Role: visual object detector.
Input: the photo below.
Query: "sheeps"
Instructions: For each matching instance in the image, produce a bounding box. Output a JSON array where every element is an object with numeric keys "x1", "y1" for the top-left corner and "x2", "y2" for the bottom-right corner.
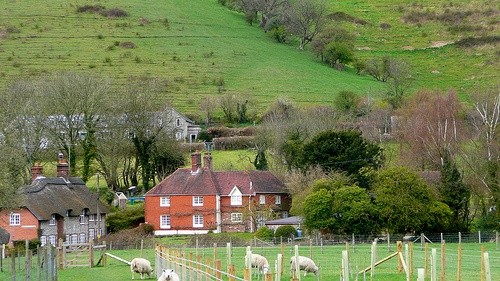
[
  {"x1": 290, "y1": 256, "x2": 319, "y2": 277},
  {"x1": 131, "y1": 257, "x2": 154, "y2": 279},
  {"x1": 245, "y1": 254, "x2": 269, "y2": 275},
  {"x1": 157, "y1": 269, "x2": 179, "y2": 281}
]
